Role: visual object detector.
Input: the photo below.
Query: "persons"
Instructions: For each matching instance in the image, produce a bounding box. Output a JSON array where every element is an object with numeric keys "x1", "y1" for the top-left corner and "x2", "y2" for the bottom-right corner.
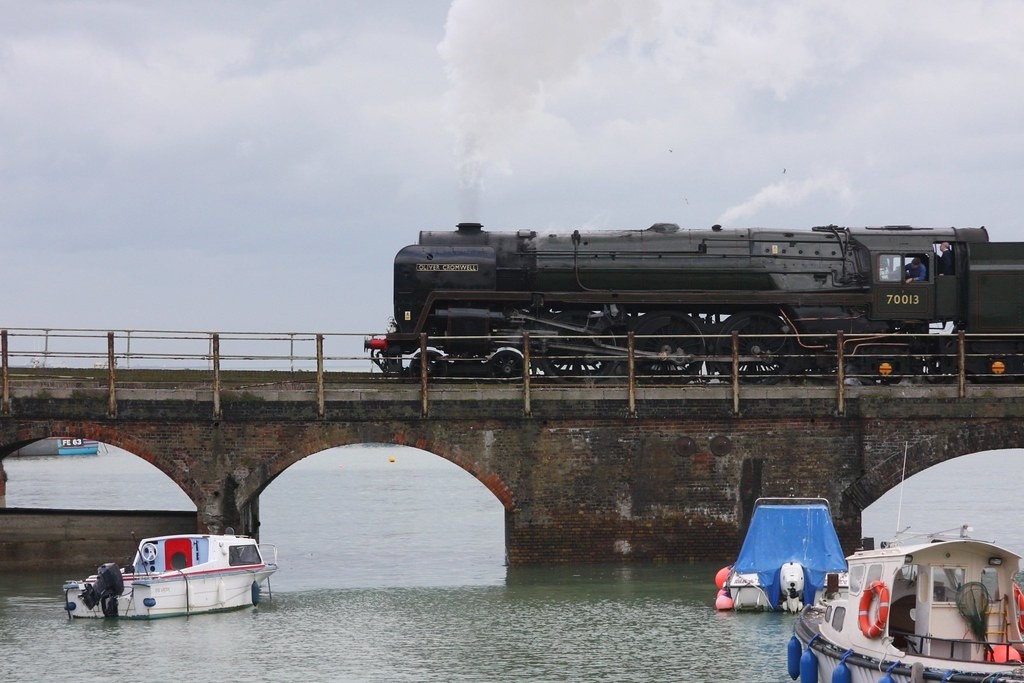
[
  {"x1": 904, "y1": 257, "x2": 926, "y2": 283},
  {"x1": 935, "y1": 242, "x2": 956, "y2": 276}
]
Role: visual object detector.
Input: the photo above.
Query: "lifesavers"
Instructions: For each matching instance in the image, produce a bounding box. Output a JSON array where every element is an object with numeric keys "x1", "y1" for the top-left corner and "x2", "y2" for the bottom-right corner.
[
  {"x1": 1014, "y1": 584, "x2": 1024, "y2": 635},
  {"x1": 859, "y1": 581, "x2": 889, "y2": 640}
]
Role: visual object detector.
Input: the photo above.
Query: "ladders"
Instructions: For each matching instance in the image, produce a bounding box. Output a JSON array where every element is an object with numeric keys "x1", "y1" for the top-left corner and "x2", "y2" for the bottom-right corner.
[{"x1": 981, "y1": 594, "x2": 1010, "y2": 651}]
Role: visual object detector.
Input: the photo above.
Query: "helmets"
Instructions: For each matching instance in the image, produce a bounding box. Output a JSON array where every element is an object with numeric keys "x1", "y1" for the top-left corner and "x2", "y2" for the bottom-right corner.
[
  {"x1": 715, "y1": 565, "x2": 733, "y2": 590},
  {"x1": 987, "y1": 644, "x2": 1022, "y2": 664}
]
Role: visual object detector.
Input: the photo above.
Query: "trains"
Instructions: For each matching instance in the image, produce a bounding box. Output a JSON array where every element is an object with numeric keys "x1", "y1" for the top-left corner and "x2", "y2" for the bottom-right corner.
[{"x1": 362, "y1": 222, "x2": 1024, "y2": 385}]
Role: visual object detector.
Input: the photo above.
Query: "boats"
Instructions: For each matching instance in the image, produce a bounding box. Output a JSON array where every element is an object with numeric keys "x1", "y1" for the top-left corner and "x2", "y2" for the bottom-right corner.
[
  {"x1": 61, "y1": 532, "x2": 277, "y2": 621},
  {"x1": 782, "y1": 441, "x2": 1024, "y2": 682},
  {"x1": 714, "y1": 497, "x2": 846, "y2": 611}
]
